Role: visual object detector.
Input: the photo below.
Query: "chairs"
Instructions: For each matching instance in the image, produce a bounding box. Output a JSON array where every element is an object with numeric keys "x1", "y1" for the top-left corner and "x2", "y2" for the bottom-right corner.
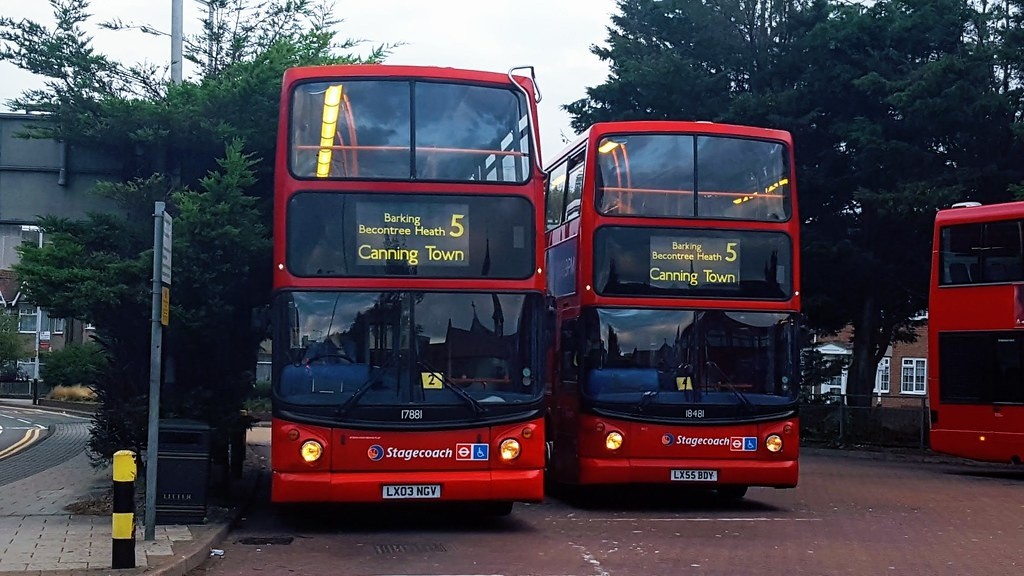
[
  {"x1": 949, "y1": 263, "x2": 971, "y2": 284},
  {"x1": 973, "y1": 265, "x2": 1003, "y2": 283}
]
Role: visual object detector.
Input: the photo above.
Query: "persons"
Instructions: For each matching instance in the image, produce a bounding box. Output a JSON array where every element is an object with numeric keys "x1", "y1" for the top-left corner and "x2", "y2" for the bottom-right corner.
[{"x1": 303, "y1": 316, "x2": 356, "y2": 364}]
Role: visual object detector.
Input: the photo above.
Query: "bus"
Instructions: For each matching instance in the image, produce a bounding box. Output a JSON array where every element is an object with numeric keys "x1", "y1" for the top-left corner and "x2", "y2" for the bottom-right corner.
[
  {"x1": 541, "y1": 118, "x2": 800, "y2": 498},
  {"x1": 271, "y1": 61, "x2": 547, "y2": 515},
  {"x1": 927, "y1": 199, "x2": 1024, "y2": 468}
]
